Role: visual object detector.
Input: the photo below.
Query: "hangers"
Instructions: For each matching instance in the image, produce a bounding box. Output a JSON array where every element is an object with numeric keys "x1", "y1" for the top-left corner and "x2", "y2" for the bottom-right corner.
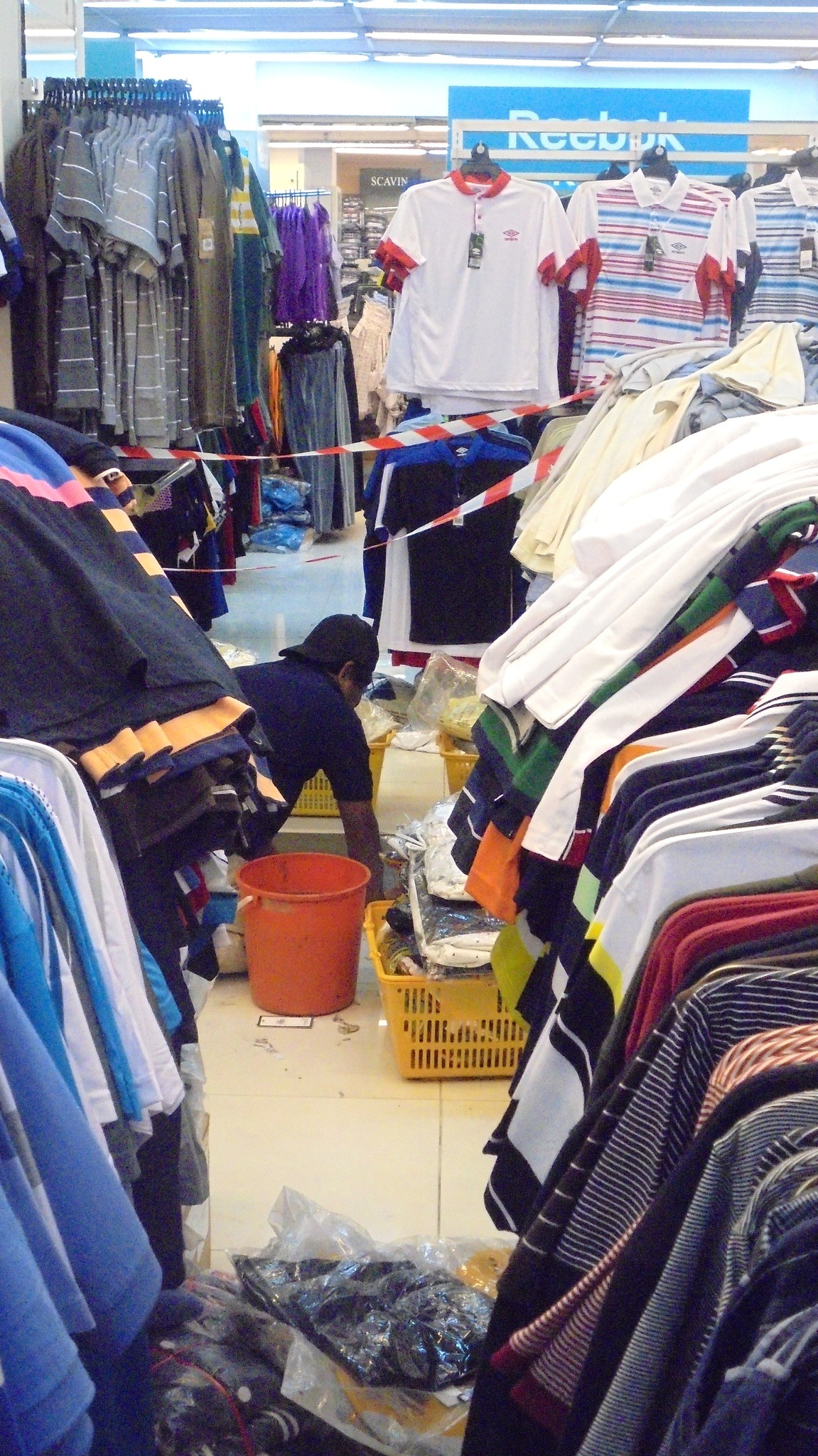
[
  {"x1": 0, "y1": 75, "x2": 818, "y2": 529},
  {"x1": 578, "y1": 581, "x2": 818, "y2": 1456}
]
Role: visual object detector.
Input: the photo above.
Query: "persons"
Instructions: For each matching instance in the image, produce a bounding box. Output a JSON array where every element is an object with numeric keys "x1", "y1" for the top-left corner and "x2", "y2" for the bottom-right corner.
[{"x1": 229, "y1": 614, "x2": 387, "y2": 908}]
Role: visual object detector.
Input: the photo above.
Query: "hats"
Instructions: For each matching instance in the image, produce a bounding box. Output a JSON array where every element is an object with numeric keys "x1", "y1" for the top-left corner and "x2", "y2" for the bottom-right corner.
[{"x1": 278, "y1": 613, "x2": 379, "y2": 668}]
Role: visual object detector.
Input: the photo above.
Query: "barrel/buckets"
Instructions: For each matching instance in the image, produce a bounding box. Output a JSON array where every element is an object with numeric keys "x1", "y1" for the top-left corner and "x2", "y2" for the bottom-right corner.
[{"x1": 235, "y1": 852, "x2": 372, "y2": 1017}]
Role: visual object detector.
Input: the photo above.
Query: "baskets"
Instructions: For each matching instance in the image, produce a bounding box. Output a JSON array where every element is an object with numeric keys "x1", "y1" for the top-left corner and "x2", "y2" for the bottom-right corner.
[
  {"x1": 364, "y1": 898, "x2": 532, "y2": 1080},
  {"x1": 441, "y1": 750, "x2": 484, "y2": 794},
  {"x1": 291, "y1": 729, "x2": 395, "y2": 819}
]
